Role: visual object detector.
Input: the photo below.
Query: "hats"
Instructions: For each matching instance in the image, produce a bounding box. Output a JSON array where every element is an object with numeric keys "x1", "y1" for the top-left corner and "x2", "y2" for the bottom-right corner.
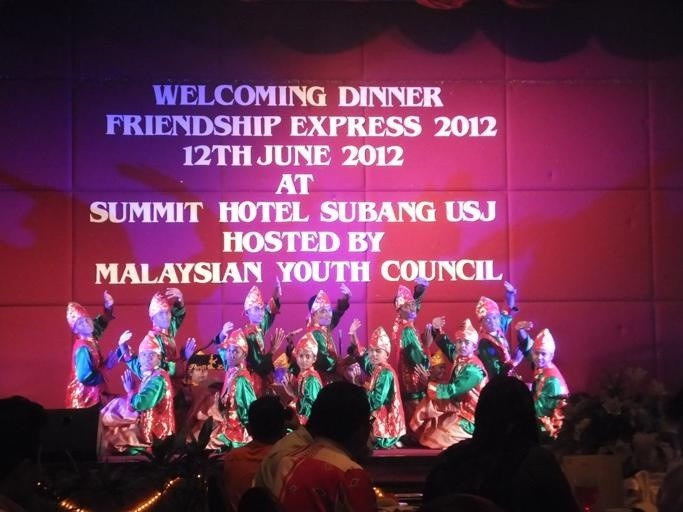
[
  {"x1": 395, "y1": 284, "x2": 415, "y2": 310},
  {"x1": 310, "y1": 289, "x2": 332, "y2": 314},
  {"x1": 295, "y1": 331, "x2": 320, "y2": 356},
  {"x1": 67, "y1": 302, "x2": 88, "y2": 329},
  {"x1": 455, "y1": 297, "x2": 499, "y2": 344},
  {"x1": 224, "y1": 329, "x2": 247, "y2": 354},
  {"x1": 243, "y1": 286, "x2": 265, "y2": 311},
  {"x1": 368, "y1": 328, "x2": 390, "y2": 355},
  {"x1": 531, "y1": 328, "x2": 555, "y2": 354},
  {"x1": 138, "y1": 292, "x2": 171, "y2": 355}
]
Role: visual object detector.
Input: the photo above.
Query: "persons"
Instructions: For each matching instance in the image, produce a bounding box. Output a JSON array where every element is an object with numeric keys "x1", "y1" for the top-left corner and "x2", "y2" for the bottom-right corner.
[
  {"x1": 655, "y1": 465, "x2": 683, "y2": 512},
  {"x1": 57, "y1": 274, "x2": 582, "y2": 512},
  {"x1": 0, "y1": 392, "x2": 50, "y2": 512}
]
